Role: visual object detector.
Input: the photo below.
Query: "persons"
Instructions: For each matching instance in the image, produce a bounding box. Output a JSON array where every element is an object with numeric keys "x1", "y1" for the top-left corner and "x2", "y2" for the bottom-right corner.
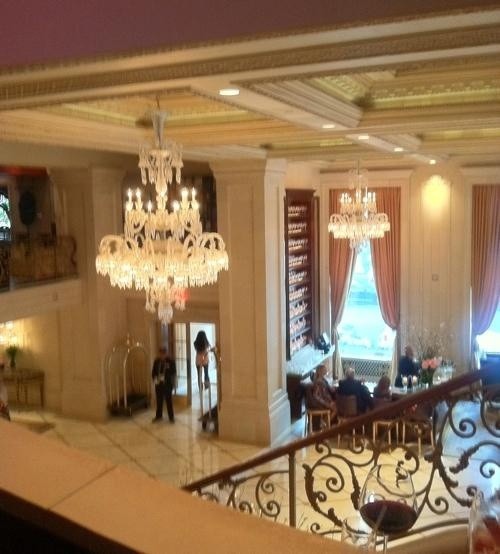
[
  {"x1": 307, "y1": 365, "x2": 338, "y2": 430},
  {"x1": 374, "y1": 375, "x2": 393, "y2": 398},
  {"x1": 334, "y1": 367, "x2": 371, "y2": 418},
  {"x1": 151, "y1": 345, "x2": 177, "y2": 424},
  {"x1": 395, "y1": 344, "x2": 431, "y2": 387},
  {"x1": 193, "y1": 330, "x2": 211, "y2": 392}
]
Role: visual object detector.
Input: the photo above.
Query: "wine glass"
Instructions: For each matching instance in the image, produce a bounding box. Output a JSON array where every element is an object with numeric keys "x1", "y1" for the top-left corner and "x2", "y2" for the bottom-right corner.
[{"x1": 358, "y1": 464, "x2": 419, "y2": 554}]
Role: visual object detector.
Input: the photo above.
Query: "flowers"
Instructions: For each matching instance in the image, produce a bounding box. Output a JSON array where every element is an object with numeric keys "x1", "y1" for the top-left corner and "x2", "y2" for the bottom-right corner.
[{"x1": 407, "y1": 320, "x2": 455, "y2": 382}]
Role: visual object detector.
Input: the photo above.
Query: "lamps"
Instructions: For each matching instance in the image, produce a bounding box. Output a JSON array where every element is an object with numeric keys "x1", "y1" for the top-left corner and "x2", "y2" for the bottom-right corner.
[
  {"x1": 326, "y1": 160, "x2": 391, "y2": 254},
  {"x1": 94, "y1": 93, "x2": 229, "y2": 326}
]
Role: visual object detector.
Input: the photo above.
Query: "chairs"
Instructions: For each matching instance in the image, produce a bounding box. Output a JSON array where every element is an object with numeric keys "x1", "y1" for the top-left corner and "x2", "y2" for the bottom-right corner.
[{"x1": 303, "y1": 384, "x2": 435, "y2": 460}]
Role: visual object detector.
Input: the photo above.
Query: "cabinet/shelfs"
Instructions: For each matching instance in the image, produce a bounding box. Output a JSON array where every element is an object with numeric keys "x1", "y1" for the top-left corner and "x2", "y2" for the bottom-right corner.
[{"x1": 282, "y1": 187, "x2": 317, "y2": 358}]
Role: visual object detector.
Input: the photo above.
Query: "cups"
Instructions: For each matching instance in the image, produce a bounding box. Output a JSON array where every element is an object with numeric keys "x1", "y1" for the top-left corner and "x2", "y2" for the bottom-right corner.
[
  {"x1": 341, "y1": 516, "x2": 377, "y2": 554},
  {"x1": 468, "y1": 487, "x2": 500, "y2": 554}
]
